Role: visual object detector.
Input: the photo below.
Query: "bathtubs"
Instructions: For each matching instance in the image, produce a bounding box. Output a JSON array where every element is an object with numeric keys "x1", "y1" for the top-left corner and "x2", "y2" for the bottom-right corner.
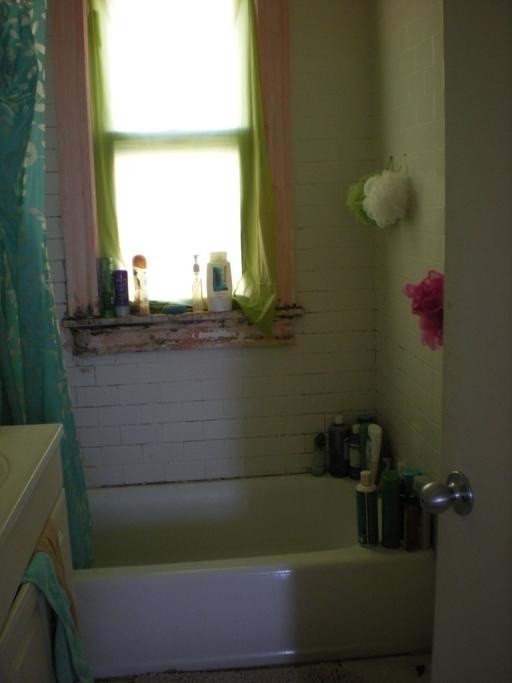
[{"x1": 72, "y1": 469, "x2": 436, "y2": 682}]
